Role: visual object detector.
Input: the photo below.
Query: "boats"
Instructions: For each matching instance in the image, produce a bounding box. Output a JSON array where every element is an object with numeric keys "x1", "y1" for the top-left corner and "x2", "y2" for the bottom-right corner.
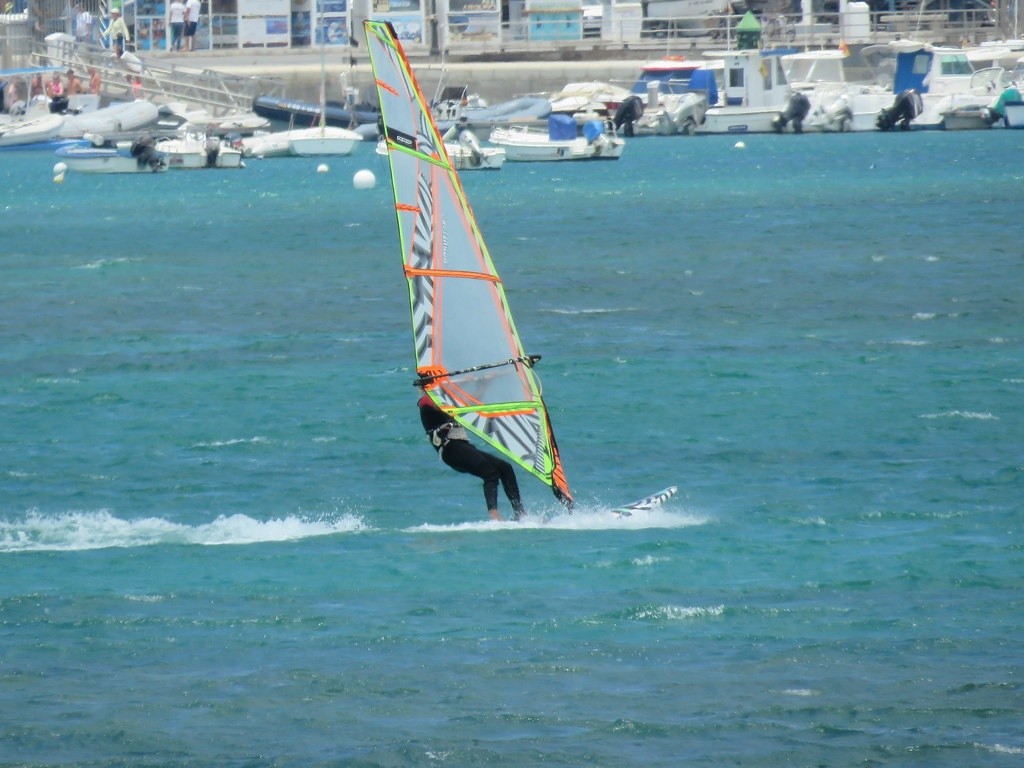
[
  {"x1": 54, "y1": 132, "x2": 172, "y2": 172},
  {"x1": 252, "y1": 84, "x2": 552, "y2": 133},
  {"x1": 154, "y1": 133, "x2": 243, "y2": 170},
  {"x1": 549, "y1": 33, "x2": 1024, "y2": 136},
  {"x1": 0, "y1": 95, "x2": 270, "y2": 147},
  {"x1": 489, "y1": 115, "x2": 624, "y2": 161},
  {"x1": 376, "y1": 137, "x2": 505, "y2": 170}
]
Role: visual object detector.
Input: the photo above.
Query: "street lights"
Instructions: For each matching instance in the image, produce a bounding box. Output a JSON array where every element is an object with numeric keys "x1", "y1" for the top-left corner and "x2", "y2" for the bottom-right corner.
[{"x1": 237, "y1": 1, "x2": 364, "y2": 157}]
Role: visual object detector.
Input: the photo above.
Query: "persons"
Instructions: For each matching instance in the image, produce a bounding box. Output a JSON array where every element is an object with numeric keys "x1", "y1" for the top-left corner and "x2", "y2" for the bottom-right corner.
[
  {"x1": 165, "y1": 0, "x2": 204, "y2": 52},
  {"x1": 0, "y1": 0, "x2": 141, "y2": 116},
  {"x1": 418, "y1": 379, "x2": 529, "y2": 525}
]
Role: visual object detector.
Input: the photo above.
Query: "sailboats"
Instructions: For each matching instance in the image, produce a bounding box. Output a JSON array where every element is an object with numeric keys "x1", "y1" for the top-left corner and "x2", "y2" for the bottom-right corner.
[{"x1": 363, "y1": 22, "x2": 679, "y2": 523}]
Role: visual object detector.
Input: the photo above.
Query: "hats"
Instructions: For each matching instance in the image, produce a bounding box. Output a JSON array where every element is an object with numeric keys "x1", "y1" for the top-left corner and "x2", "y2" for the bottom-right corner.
[{"x1": 110, "y1": 7, "x2": 119, "y2": 13}]
